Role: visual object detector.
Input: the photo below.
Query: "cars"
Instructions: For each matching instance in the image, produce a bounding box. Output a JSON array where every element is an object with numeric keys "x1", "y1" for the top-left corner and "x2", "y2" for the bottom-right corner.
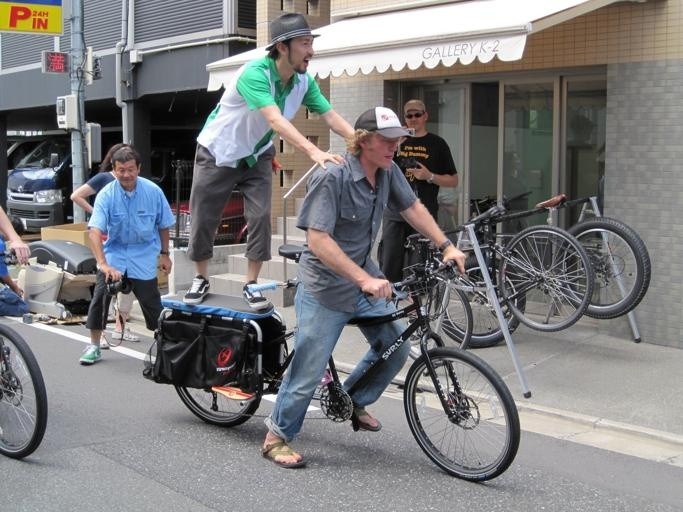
[{"x1": 167, "y1": 182, "x2": 249, "y2": 247}]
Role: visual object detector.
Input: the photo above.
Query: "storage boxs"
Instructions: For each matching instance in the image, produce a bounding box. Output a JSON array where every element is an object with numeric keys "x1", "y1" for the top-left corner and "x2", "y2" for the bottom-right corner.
[{"x1": 40, "y1": 222, "x2": 91, "y2": 248}]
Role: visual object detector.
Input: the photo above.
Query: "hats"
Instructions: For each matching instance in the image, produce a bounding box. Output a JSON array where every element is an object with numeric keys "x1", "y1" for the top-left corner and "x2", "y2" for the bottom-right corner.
[
  {"x1": 354, "y1": 106, "x2": 411, "y2": 139},
  {"x1": 403, "y1": 99, "x2": 425, "y2": 113},
  {"x1": 264, "y1": 12, "x2": 320, "y2": 50}
]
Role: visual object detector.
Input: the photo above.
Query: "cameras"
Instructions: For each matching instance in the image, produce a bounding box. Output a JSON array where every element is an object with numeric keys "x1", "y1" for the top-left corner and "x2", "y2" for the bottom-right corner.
[
  {"x1": 106, "y1": 275, "x2": 135, "y2": 294},
  {"x1": 405, "y1": 128, "x2": 415, "y2": 137}
]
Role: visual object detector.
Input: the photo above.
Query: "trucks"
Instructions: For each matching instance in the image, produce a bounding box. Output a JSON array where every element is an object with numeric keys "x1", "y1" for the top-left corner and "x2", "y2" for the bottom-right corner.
[
  {"x1": 5, "y1": 136, "x2": 59, "y2": 173},
  {"x1": 2, "y1": 136, "x2": 207, "y2": 233}
]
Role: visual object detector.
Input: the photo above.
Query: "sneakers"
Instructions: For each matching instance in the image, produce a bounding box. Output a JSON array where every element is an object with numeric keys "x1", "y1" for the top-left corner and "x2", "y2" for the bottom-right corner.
[
  {"x1": 182, "y1": 274, "x2": 210, "y2": 306},
  {"x1": 79, "y1": 345, "x2": 101, "y2": 364},
  {"x1": 99, "y1": 335, "x2": 109, "y2": 349},
  {"x1": 242, "y1": 280, "x2": 268, "y2": 311},
  {"x1": 111, "y1": 331, "x2": 140, "y2": 342},
  {"x1": 0, "y1": 360, "x2": 17, "y2": 387}
]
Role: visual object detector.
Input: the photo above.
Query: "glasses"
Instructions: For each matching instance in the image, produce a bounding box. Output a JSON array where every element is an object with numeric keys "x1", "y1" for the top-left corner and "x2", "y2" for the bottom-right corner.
[{"x1": 405, "y1": 111, "x2": 424, "y2": 118}]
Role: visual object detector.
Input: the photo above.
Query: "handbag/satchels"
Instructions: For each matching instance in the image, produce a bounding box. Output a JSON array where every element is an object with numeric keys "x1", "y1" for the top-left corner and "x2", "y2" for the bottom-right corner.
[{"x1": 143, "y1": 316, "x2": 249, "y2": 393}]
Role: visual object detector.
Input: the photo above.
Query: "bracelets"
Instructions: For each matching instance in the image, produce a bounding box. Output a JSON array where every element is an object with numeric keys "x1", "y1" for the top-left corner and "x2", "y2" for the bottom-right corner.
[
  {"x1": 439, "y1": 239, "x2": 452, "y2": 253},
  {"x1": 160, "y1": 250, "x2": 170, "y2": 256}
]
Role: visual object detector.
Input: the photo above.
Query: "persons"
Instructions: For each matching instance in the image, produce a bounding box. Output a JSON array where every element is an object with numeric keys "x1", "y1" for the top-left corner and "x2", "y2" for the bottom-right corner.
[
  {"x1": 79, "y1": 149, "x2": 175, "y2": 364},
  {"x1": 70, "y1": 144, "x2": 141, "y2": 349},
  {"x1": 378, "y1": 100, "x2": 459, "y2": 345},
  {"x1": 260, "y1": 107, "x2": 467, "y2": 469},
  {"x1": 1, "y1": 208, "x2": 30, "y2": 266},
  {"x1": 0, "y1": 215, "x2": 24, "y2": 297},
  {"x1": 182, "y1": 12, "x2": 358, "y2": 311}
]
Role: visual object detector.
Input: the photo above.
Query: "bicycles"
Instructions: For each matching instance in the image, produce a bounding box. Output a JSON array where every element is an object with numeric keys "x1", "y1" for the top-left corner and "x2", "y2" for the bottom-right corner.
[
  {"x1": 156, "y1": 244, "x2": 520, "y2": 483},
  {"x1": 393, "y1": 205, "x2": 594, "y2": 366},
  {"x1": 0, "y1": 248, "x2": 47, "y2": 459},
  {"x1": 433, "y1": 194, "x2": 652, "y2": 348}
]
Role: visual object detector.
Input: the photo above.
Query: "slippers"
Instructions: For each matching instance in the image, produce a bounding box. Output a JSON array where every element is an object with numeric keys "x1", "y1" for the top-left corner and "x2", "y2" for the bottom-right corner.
[
  {"x1": 260, "y1": 441, "x2": 307, "y2": 468},
  {"x1": 349, "y1": 407, "x2": 381, "y2": 431}
]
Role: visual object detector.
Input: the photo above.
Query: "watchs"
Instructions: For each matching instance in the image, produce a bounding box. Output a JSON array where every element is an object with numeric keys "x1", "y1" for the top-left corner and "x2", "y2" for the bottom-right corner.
[{"x1": 427, "y1": 173, "x2": 434, "y2": 184}]
